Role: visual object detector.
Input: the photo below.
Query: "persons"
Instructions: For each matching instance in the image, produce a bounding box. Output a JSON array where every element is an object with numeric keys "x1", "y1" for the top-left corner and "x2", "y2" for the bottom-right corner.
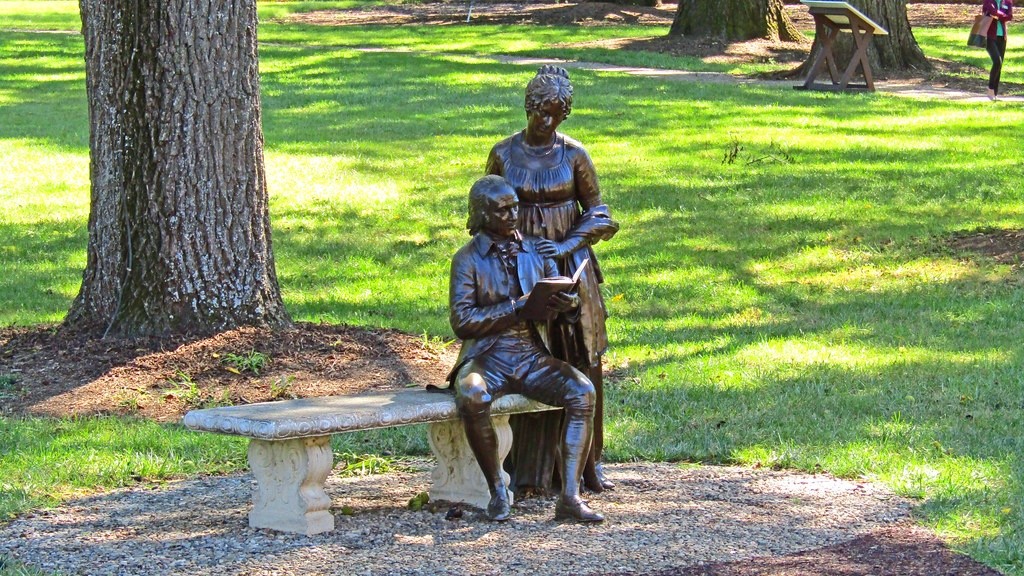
[
  {"x1": 449, "y1": 174, "x2": 610, "y2": 523},
  {"x1": 478, "y1": 65, "x2": 627, "y2": 491},
  {"x1": 981, "y1": 0, "x2": 1014, "y2": 101}
]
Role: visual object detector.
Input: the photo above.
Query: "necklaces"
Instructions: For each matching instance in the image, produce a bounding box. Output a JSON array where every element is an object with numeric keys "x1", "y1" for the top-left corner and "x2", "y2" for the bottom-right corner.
[{"x1": 518, "y1": 127, "x2": 559, "y2": 157}]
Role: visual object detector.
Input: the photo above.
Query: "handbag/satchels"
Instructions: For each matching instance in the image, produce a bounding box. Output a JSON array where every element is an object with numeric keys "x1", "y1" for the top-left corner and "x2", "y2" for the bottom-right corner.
[{"x1": 969, "y1": 8, "x2": 993, "y2": 49}]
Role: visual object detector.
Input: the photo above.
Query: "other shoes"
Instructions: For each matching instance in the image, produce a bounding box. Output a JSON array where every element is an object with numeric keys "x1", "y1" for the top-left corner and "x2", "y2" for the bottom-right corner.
[{"x1": 987, "y1": 87, "x2": 1002, "y2": 102}]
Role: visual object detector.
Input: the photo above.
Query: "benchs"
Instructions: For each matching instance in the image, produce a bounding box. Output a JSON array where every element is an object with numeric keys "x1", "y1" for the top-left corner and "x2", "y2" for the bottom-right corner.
[{"x1": 182, "y1": 383, "x2": 568, "y2": 538}]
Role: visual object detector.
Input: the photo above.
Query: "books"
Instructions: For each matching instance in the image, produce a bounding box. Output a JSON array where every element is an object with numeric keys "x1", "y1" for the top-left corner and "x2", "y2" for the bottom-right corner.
[{"x1": 516, "y1": 257, "x2": 592, "y2": 321}]
